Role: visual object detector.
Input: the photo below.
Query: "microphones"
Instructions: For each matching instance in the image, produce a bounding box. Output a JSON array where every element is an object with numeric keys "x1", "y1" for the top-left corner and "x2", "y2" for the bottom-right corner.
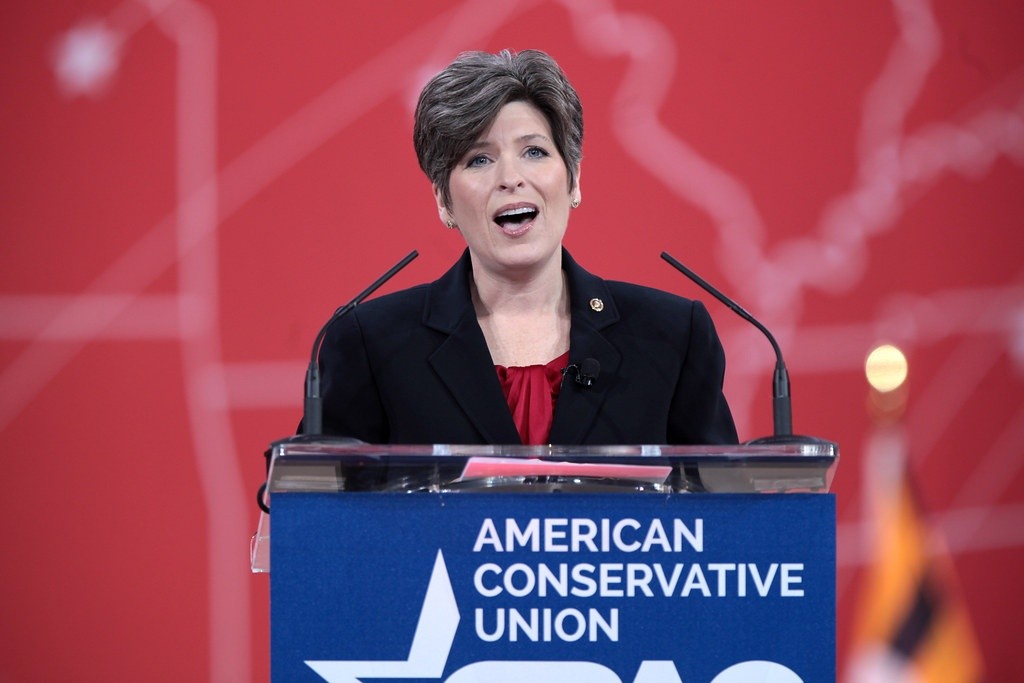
[
  {"x1": 581, "y1": 358, "x2": 601, "y2": 387},
  {"x1": 661, "y1": 251, "x2": 824, "y2": 448},
  {"x1": 269, "y1": 249, "x2": 419, "y2": 450}
]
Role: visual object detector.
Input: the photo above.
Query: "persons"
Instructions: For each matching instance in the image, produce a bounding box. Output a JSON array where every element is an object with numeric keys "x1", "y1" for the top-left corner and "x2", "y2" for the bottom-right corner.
[{"x1": 296, "y1": 47, "x2": 740, "y2": 493}]
